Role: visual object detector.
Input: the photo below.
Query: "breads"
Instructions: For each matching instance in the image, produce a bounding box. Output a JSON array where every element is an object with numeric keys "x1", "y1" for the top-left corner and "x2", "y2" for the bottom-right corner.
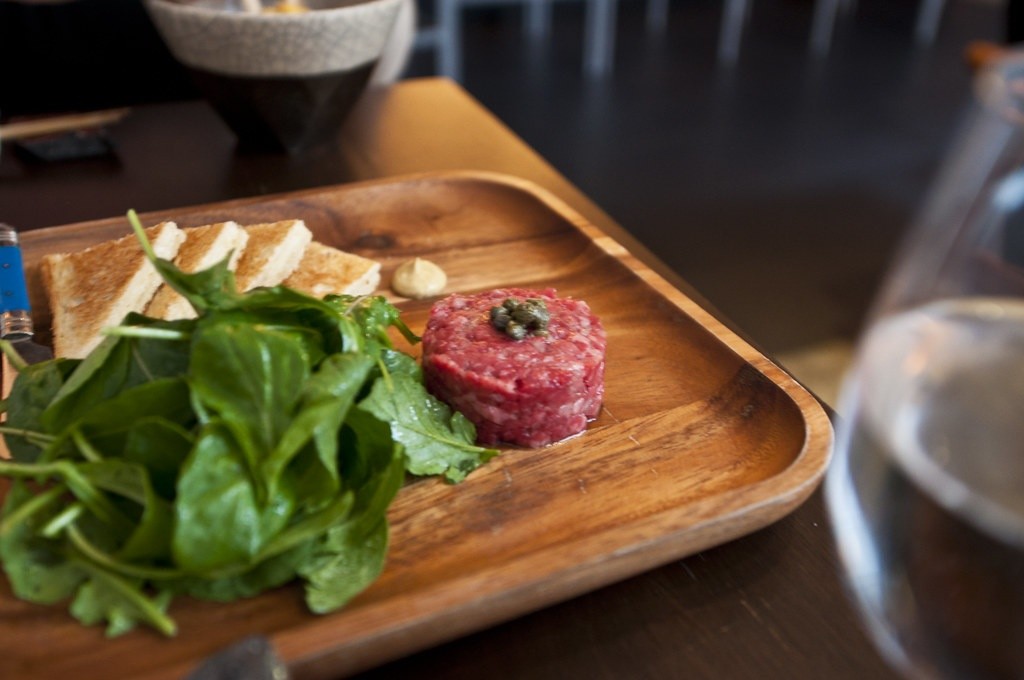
[{"x1": 39, "y1": 217, "x2": 382, "y2": 360}]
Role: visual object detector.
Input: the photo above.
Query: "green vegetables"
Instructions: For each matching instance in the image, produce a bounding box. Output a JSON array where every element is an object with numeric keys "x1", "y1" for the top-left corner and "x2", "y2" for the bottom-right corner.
[{"x1": 0, "y1": 208, "x2": 503, "y2": 638}]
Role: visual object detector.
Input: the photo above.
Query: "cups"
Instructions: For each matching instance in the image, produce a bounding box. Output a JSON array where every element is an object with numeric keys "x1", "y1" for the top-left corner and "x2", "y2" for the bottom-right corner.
[
  {"x1": 823, "y1": 58, "x2": 1024, "y2": 679},
  {"x1": 146, "y1": 0, "x2": 402, "y2": 157}
]
